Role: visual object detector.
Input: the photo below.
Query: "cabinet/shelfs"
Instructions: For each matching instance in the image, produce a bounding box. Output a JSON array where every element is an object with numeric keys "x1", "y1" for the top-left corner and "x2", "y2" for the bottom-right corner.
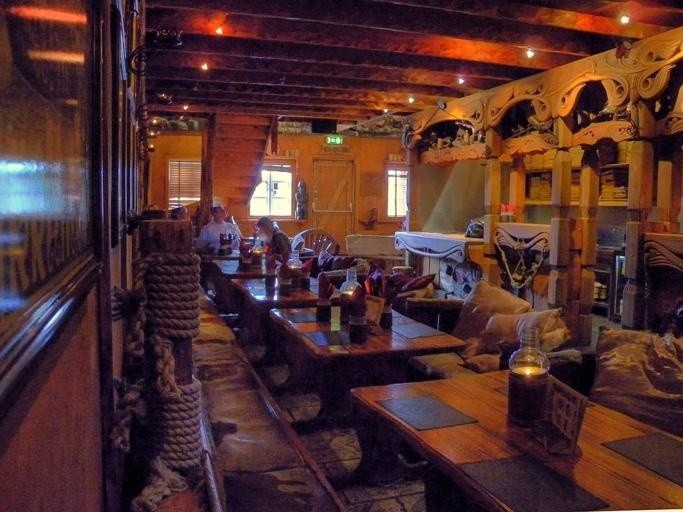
[{"x1": 508, "y1": 139, "x2": 653, "y2": 224}]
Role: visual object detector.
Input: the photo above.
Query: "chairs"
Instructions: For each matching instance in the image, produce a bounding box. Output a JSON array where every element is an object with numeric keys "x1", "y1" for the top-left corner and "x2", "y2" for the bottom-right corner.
[
  {"x1": 547, "y1": 345, "x2": 596, "y2": 398},
  {"x1": 299, "y1": 228, "x2": 339, "y2": 255},
  {"x1": 288, "y1": 235, "x2": 314, "y2": 256},
  {"x1": 299, "y1": 256, "x2": 385, "y2": 288},
  {"x1": 403, "y1": 298, "x2": 595, "y2": 397}
]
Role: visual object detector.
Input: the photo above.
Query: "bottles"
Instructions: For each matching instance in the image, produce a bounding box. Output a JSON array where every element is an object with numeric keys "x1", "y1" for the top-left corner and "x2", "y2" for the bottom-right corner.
[
  {"x1": 265, "y1": 250, "x2": 310, "y2": 295},
  {"x1": 219, "y1": 232, "x2": 263, "y2": 269},
  {"x1": 507, "y1": 326, "x2": 551, "y2": 427},
  {"x1": 463, "y1": 130, "x2": 469, "y2": 146},
  {"x1": 316, "y1": 267, "x2": 393, "y2": 344}
]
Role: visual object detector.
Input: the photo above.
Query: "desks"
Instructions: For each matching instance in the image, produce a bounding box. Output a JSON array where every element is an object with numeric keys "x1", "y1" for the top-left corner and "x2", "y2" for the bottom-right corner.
[
  {"x1": 268, "y1": 304, "x2": 466, "y2": 430},
  {"x1": 347, "y1": 368, "x2": 682, "y2": 512},
  {"x1": 203, "y1": 244, "x2": 242, "y2": 288},
  {"x1": 229, "y1": 275, "x2": 318, "y2": 344},
  {"x1": 210, "y1": 259, "x2": 270, "y2": 311},
  {"x1": 328, "y1": 271, "x2": 464, "y2": 334}
]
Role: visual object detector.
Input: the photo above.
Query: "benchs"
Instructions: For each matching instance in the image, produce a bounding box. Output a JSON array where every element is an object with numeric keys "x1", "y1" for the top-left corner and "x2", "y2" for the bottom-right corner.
[{"x1": 345, "y1": 233, "x2": 405, "y2": 273}]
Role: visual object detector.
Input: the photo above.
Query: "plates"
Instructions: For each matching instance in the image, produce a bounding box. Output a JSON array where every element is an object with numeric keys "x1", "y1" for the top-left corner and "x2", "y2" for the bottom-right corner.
[{"x1": 593, "y1": 281, "x2": 608, "y2": 300}]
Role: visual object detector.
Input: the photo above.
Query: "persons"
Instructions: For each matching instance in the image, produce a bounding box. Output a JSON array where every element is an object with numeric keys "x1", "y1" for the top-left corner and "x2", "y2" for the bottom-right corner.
[
  {"x1": 197, "y1": 204, "x2": 241, "y2": 252},
  {"x1": 294, "y1": 179, "x2": 308, "y2": 220},
  {"x1": 258, "y1": 217, "x2": 290, "y2": 253},
  {"x1": 173, "y1": 208, "x2": 189, "y2": 218}
]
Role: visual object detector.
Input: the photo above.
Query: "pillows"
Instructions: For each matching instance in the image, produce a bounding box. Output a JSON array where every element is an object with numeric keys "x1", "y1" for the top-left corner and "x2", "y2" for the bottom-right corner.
[
  {"x1": 316, "y1": 248, "x2": 332, "y2": 272},
  {"x1": 477, "y1": 309, "x2": 561, "y2": 352},
  {"x1": 590, "y1": 325, "x2": 683, "y2": 438},
  {"x1": 332, "y1": 255, "x2": 353, "y2": 271},
  {"x1": 399, "y1": 273, "x2": 438, "y2": 293},
  {"x1": 292, "y1": 234, "x2": 304, "y2": 253},
  {"x1": 450, "y1": 279, "x2": 530, "y2": 356}
]
